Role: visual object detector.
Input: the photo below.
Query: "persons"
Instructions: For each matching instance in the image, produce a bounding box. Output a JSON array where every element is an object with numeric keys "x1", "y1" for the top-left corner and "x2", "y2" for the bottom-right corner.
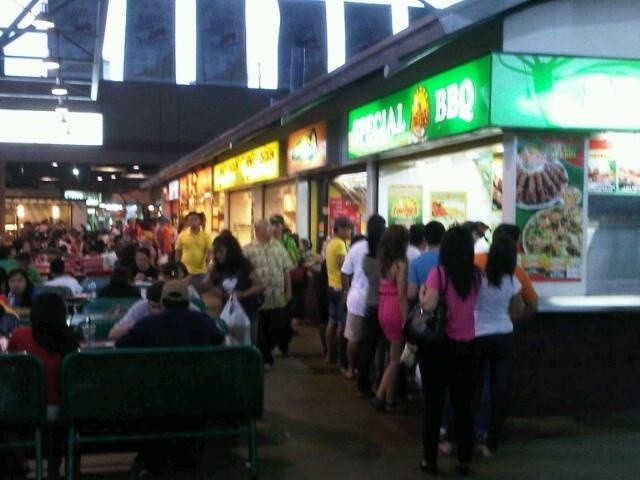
[{"x1": 1, "y1": 212, "x2": 538, "y2": 480}]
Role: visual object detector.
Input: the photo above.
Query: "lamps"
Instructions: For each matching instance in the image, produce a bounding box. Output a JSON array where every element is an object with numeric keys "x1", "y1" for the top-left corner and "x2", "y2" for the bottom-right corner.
[
  {"x1": 51, "y1": 68, "x2": 67, "y2": 95},
  {"x1": 43, "y1": 30, "x2": 59, "y2": 70},
  {"x1": 32, "y1": 1, "x2": 56, "y2": 28},
  {"x1": 54, "y1": 96, "x2": 67, "y2": 115}
]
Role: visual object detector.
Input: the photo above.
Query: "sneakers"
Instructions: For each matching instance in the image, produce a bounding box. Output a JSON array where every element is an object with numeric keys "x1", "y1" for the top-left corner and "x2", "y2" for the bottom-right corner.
[{"x1": 420, "y1": 459, "x2": 438, "y2": 472}]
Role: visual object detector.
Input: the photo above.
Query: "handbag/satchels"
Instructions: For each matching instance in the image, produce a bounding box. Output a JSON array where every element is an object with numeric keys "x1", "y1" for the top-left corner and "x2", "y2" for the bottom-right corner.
[{"x1": 403, "y1": 299, "x2": 446, "y2": 351}]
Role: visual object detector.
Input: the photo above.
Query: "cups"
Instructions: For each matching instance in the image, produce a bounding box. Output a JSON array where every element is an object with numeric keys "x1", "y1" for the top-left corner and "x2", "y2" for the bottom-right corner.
[{"x1": 82, "y1": 324, "x2": 97, "y2": 347}]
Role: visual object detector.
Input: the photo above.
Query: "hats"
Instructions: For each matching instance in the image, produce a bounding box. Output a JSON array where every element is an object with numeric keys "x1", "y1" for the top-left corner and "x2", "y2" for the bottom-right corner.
[
  {"x1": 14, "y1": 253, "x2": 31, "y2": 260},
  {"x1": 162, "y1": 280, "x2": 190, "y2": 302}
]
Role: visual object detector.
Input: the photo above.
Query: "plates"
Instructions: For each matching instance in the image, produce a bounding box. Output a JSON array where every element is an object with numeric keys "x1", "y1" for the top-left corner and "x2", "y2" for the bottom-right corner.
[
  {"x1": 517, "y1": 152, "x2": 570, "y2": 211},
  {"x1": 522, "y1": 206, "x2": 585, "y2": 259}
]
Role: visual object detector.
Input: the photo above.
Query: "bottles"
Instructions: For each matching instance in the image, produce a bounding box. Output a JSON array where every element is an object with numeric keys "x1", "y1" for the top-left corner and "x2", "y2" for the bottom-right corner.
[
  {"x1": 7, "y1": 287, "x2": 17, "y2": 310},
  {"x1": 88, "y1": 279, "x2": 97, "y2": 301}
]
